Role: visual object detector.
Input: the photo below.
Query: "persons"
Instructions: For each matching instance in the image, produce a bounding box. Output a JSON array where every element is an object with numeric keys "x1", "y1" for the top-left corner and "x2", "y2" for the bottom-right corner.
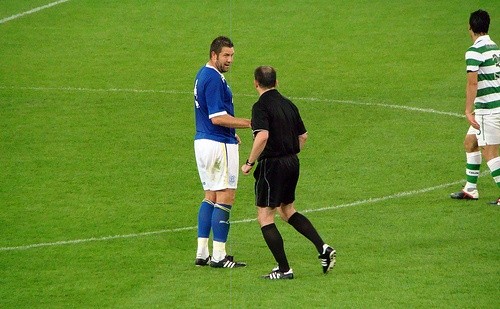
[
  {"x1": 193, "y1": 36, "x2": 251, "y2": 268},
  {"x1": 240, "y1": 65, "x2": 337, "y2": 280},
  {"x1": 450, "y1": 9, "x2": 500, "y2": 207}
]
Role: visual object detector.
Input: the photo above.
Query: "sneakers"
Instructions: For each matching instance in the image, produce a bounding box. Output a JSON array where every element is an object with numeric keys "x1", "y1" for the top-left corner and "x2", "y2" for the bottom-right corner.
[
  {"x1": 262, "y1": 267, "x2": 293, "y2": 281},
  {"x1": 209, "y1": 255, "x2": 246, "y2": 269},
  {"x1": 450, "y1": 188, "x2": 479, "y2": 200},
  {"x1": 488, "y1": 197, "x2": 500, "y2": 205},
  {"x1": 317, "y1": 244, "x2": 337, "y2": 274},
  {"x1": 195, "y1": 255, "x2": 212, "y2": 267}
]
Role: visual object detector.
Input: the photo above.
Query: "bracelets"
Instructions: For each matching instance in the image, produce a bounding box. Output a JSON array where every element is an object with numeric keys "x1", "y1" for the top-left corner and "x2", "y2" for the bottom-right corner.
[{"x1": 246, "y1": 158, "x2": 255, "y2": 166}]
